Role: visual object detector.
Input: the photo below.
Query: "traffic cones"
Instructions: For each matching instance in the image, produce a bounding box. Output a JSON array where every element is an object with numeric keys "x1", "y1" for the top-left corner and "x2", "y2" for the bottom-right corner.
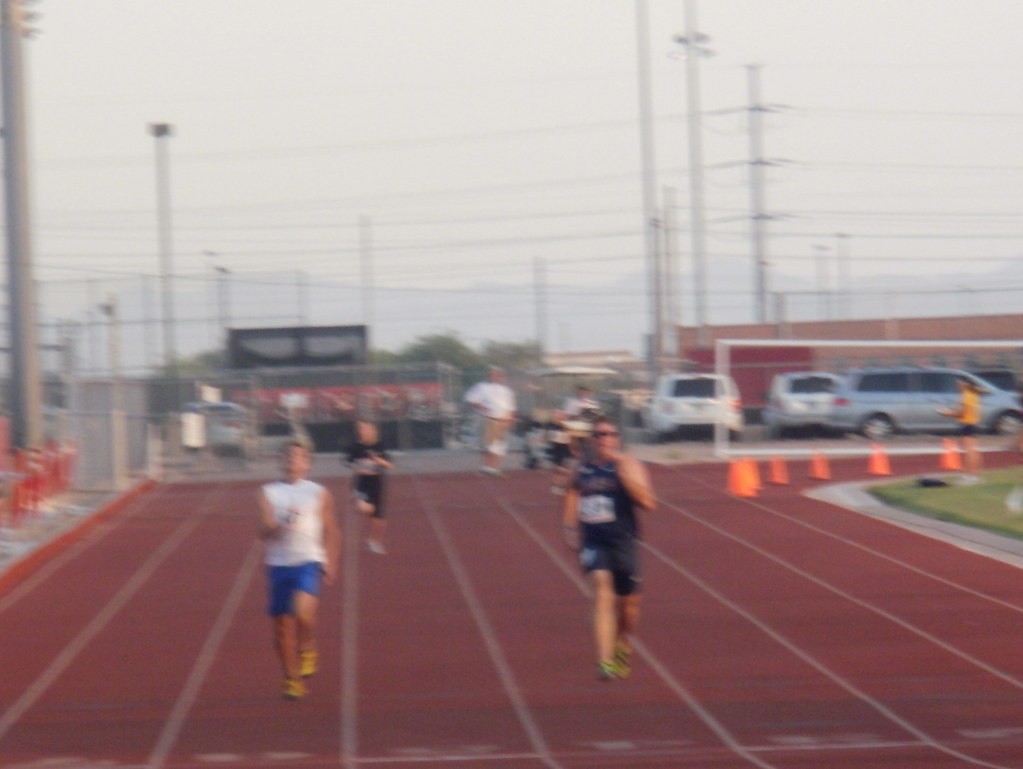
[{"x1": 722, "y1": 437, "x2": 981, "y2": 498}]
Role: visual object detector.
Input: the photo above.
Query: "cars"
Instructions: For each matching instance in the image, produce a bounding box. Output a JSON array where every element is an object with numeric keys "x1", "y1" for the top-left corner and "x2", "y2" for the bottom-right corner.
[
  {"x1": 826, "y1": 366, "x2": 1022, "y2": 440},
  {"x1": 644, "y1": 370, "x2": 746, "y2": 444},
  {"x1": 756, "y1": 368, "x2": 855, "y2": 441}
]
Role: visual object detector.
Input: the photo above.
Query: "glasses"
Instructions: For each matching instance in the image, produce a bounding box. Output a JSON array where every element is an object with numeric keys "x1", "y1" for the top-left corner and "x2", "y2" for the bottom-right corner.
[{"x1": 592, "y1": 429, "x2": 620, "y2": 440}]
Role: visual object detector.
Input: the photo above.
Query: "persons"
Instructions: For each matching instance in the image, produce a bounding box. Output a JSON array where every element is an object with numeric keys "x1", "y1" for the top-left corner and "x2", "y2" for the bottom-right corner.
[
  {"x1": 936, "y1": 375, "x2": 984, "y2": 445},
  {"x1": 461, "y1": 368, "x2": 615, "y2": 522},
  {"x1": 343, "y1": 417, "x2": 393, "y2": 558},
  {"x1": 562, "y1": 419, "x2": 658, "y2": 684},
  {"x1": 253, "y1": 444, "x2": 342, "y2": 701}
]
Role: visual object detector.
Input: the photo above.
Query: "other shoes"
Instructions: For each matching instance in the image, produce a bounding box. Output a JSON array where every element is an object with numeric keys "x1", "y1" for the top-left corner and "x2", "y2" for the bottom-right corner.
[
  {"x1": 281, "y1": 680, "x2": 306, "y2": 700},
  {"x1": 600, "y1": 660, "x2": 617, "y2": 682},
  {"x1": 301, "y1": 649, "x2": 319, "y2": 676},
  {"x1": 615, "y1": 648, "x2": 633, "y2": 679}
]
